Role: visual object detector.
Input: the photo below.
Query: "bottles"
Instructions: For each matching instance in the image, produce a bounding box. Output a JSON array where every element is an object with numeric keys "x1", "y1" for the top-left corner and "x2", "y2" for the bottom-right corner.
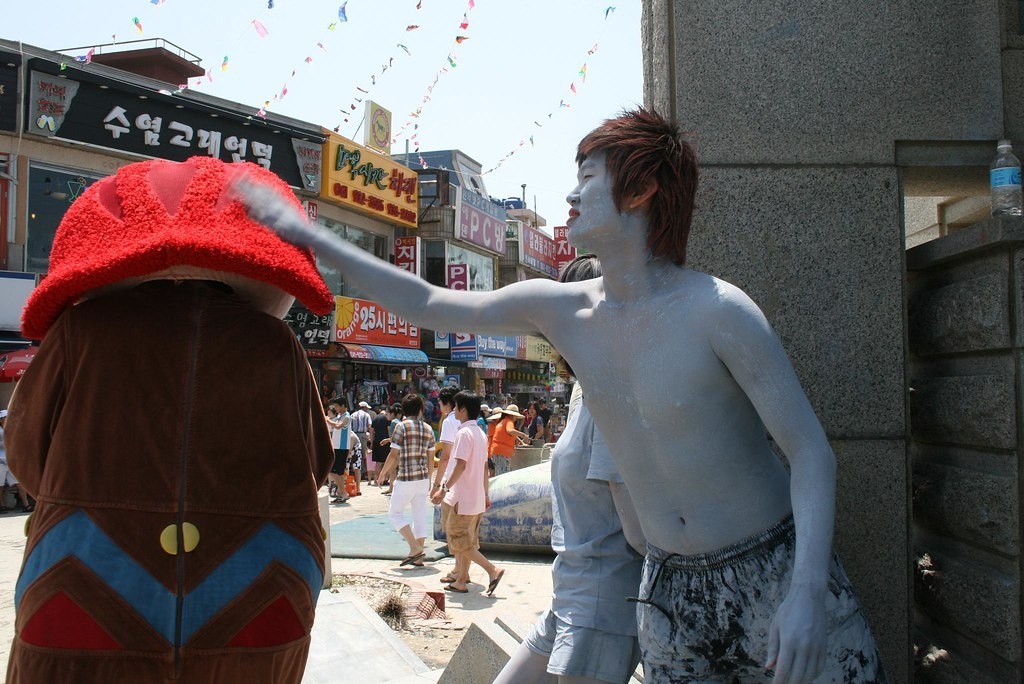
[{"x1": 989, "y1": 139, "x2": 1024, "y2": 218}]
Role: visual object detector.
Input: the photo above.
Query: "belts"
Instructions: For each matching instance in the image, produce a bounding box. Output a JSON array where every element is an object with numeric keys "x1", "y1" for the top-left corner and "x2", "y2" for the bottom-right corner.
[{"x1": 354, "y1": 432, "x2": 364, "y2": 434}]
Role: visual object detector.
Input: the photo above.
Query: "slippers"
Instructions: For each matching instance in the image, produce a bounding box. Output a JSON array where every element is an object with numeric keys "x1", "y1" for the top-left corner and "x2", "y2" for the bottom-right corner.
[
  {"x1": 330, "y1": 501, "x2": 346, "y2": 504},
  {"x1": 444, "y1": 584, "x2": 468, "y2": 593},
  {"x1": 447, "y1": 571, "x2": 457, "y2": 577},
  {"x1": 440, "y1": 576, "x2": 471, "y2": 583},
  {"x1": 400, "y1": 553, "x2": 426, "y2": 567},
  {"x1": 488, "y1": 569, "x2": 505, "y2": 596},
  {"x1": 410, "y1": 562, "x2": 424, "y2": 566},
  {"x1": 334, "y1": 498, "x2": 350, "y2": 504}
]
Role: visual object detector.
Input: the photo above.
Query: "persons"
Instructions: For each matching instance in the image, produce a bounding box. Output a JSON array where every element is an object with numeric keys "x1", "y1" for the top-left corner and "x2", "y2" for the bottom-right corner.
[
  {"x1": 492, "y1": 253, "x2": 644, "y2": 684},
  {"x1": 232, "y1": 105, "x2": 888, "y2": 684},
  {"x1": 0, "y1": 410, "x2": 35, "y2": 514},
  {"x1": 326, "y1": 385, "x2": 552, "y2": 598}
]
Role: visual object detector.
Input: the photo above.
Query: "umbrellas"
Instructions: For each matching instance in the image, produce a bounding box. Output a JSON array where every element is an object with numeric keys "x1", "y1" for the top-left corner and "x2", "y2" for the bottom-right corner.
[{"x1": 0, "y1": 346, "x2": 39, "y2": 383}]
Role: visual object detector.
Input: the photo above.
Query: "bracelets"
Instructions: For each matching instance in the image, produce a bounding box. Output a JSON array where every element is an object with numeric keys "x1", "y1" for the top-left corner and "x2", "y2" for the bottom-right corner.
[
  {"x1": 433, "y1": 484, "x2": 441, "y2": 490},
  {"x1": 442, "y1": 484, "x2": 450, "y2": 492}
]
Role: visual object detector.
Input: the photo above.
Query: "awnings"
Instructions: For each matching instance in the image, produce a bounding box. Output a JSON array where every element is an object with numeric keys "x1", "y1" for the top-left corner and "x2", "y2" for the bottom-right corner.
[{"x1": 340, "y1": 343, "x2": 429, "y2": 364}]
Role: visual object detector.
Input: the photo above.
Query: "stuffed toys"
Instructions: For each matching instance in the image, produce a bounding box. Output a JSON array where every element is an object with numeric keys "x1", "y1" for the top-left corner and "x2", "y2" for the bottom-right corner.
[{"x1": 5, "y1": 155, "x2": 338, "y2": 684}]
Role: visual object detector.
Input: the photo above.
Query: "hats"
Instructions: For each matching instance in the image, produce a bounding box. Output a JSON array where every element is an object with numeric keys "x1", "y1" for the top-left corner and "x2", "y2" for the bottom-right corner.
[
  {"x1": 359, "y1": 401, "x2": 372, "y2": 409},
  {"x1": 485, "y1": 407, "x2": 504, "y2": 420},
  {"x1": 480, "y1": 404, "x2": 493, "y2": 412},
  {"x1": 497, "y1": 404, "x2": 525, "y2": 419},
  {"x1": 0, "y1": 410, "x2": 7, "y2": 418}
]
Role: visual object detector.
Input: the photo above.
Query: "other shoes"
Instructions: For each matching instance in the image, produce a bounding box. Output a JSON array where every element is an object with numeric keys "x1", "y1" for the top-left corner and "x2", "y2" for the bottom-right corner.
[
  {"x1": 357, "y1": 491, "x2": 362, "y2": 496},
  {"x1": 381, "y1": 491, "x2": 389, "y2": 494},
  {"x1": 385, "y1": 493, "x2": 392, "y2": 496},
  {"x1": 22, "y1": 505, "x2": 34, "y2": 512},
  {"x1": 0, "y1": 506, "x2": 8, "y2": 514}
]
